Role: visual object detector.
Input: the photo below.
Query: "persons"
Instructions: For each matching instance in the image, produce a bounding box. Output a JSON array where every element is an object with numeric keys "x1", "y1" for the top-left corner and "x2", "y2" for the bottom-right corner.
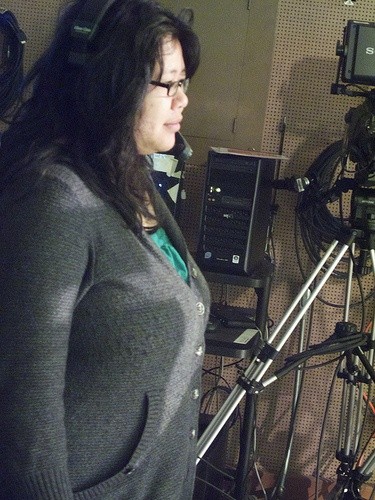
[{"x1": 0, "y1": 0, "x2": 211, "y2": 500}]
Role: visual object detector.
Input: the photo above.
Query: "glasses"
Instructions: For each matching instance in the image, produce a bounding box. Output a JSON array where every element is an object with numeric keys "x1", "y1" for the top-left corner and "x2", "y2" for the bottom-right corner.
[{"x1": 150, "y1": 78, "x2": 189, "y2": 97}]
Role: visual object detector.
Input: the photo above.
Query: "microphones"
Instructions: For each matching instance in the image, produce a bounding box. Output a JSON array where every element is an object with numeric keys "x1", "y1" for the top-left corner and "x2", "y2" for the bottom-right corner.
[{"x1": 158, "y1": 131, "x2": 193, "y2": 161}]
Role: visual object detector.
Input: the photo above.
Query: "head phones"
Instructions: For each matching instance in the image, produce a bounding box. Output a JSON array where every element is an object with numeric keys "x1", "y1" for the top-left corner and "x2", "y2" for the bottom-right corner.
[{"x1": 44, "y1": 0, "x2": 121, "y2": 123}]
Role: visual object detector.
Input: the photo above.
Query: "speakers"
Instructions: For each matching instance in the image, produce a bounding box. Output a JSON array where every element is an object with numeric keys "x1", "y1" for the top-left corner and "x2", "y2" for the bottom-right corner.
[{"x1": 195, "y1": 153, "x2": 276, "y2": 275}]
